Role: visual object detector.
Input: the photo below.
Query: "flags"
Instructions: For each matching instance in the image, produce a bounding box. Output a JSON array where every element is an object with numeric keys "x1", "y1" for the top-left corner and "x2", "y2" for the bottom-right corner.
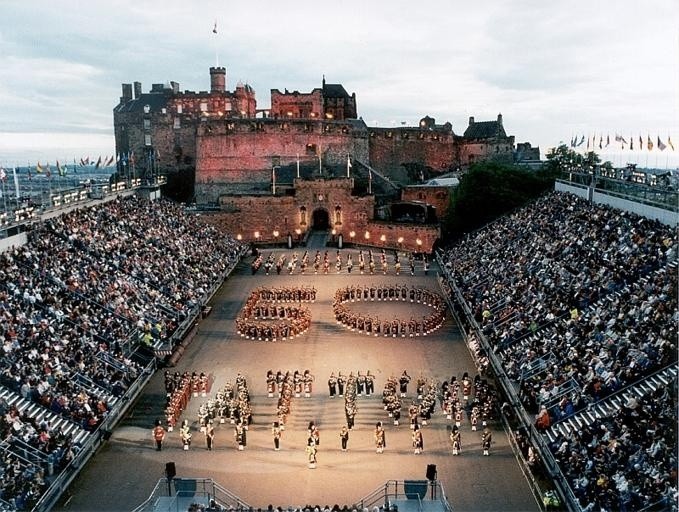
[
  {"x1": 0, "y1": 149, "x2": 161, "y2": 184},
  {"x1": 570, "y1": 132, "x2": 675, "y2": 150}
]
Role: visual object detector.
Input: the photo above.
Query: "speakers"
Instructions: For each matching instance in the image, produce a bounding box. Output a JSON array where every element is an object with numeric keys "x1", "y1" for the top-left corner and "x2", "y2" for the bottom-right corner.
[
  {"x1": 426, "y1": 464, "x2": 436, "y2": 480},
  {"x1": 166, "y1": 462, "x2": 176, "y2": 476}
]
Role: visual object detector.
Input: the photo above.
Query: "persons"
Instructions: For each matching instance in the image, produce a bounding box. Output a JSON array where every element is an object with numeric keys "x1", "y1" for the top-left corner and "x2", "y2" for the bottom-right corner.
[{"x1": 0, "y1": 190, "x2": 679, "y2": 512}]
